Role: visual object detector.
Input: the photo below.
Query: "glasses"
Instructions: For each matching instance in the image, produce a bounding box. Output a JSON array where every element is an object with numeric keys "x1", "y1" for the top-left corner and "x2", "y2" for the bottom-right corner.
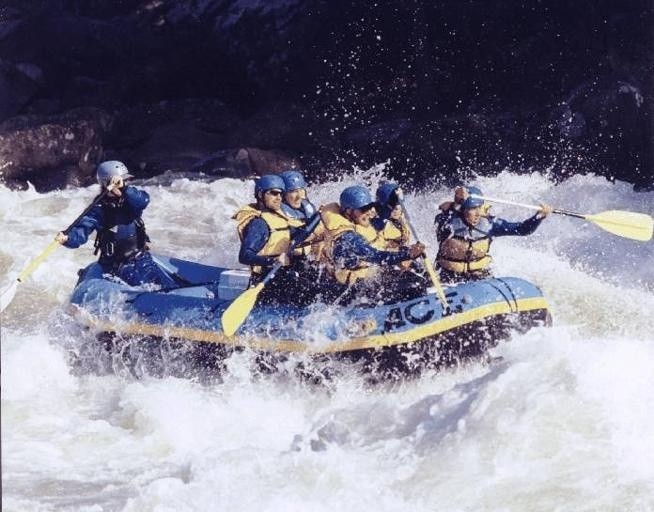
[{"x1": 265, "y1": 190, "x2": 283, "y2": 198}]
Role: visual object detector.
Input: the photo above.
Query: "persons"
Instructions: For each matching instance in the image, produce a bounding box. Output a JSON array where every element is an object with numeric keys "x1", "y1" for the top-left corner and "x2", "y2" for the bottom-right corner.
[
  {"x1": 232, "y1": 175, "x2": 323, "y2": 306},
  {"x1": 312, "y1": 186, "x2": 426, "y2": 309},
  {"x1": 375, "y1": 184, "x2": 433, "y2": 287},
  {"x1": 279, "y1": 171, "x2": 318, "y2": 282},
  {"x1": 54, "y1": 161, "x2": 181, "y2": 291},
  {"x1": 434, "y1": 187, "x2": 554, "y2": 283}
]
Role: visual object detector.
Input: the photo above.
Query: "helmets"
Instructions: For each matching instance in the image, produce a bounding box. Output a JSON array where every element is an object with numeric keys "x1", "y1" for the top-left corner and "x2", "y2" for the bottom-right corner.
[
  {"x1": 338, "y1": 185, "x2": 375, "y2": 212},
  {"x1": 454, "y1": 185, "x2": 484, "y2": 209},
  {"x1": 375, "y1": 183, "x2": 402, "y2": 204},
  {"x1": 279, "y1": 170, "x2": 306, "y2": 192},
  {"x1": 96, "y1": 160, "x2": 135, "y2": 189},
  {"x1": 254, "y1": 174, "x2": 286, "y2": 199}
]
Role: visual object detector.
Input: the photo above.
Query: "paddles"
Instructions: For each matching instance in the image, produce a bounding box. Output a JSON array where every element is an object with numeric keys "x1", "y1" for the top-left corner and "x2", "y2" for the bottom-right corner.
[
  {"x1": 1, "y1": 176, "x2": 120, "y2": 315},
  {"x1": 220, "y1": 213, "x2": 320, "y2": 338},
  {"x1": 467, "y1": 193, "x2": 653, "y2": 241},
  {"x1": 399, "y1": 197, "x2": 450, "y2": 309}
]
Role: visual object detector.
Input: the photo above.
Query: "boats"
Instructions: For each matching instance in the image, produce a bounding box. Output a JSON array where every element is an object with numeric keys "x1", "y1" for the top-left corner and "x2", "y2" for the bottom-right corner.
[{"x1": 65, "y1": 251, "x2": 552, "y2": 387}]
